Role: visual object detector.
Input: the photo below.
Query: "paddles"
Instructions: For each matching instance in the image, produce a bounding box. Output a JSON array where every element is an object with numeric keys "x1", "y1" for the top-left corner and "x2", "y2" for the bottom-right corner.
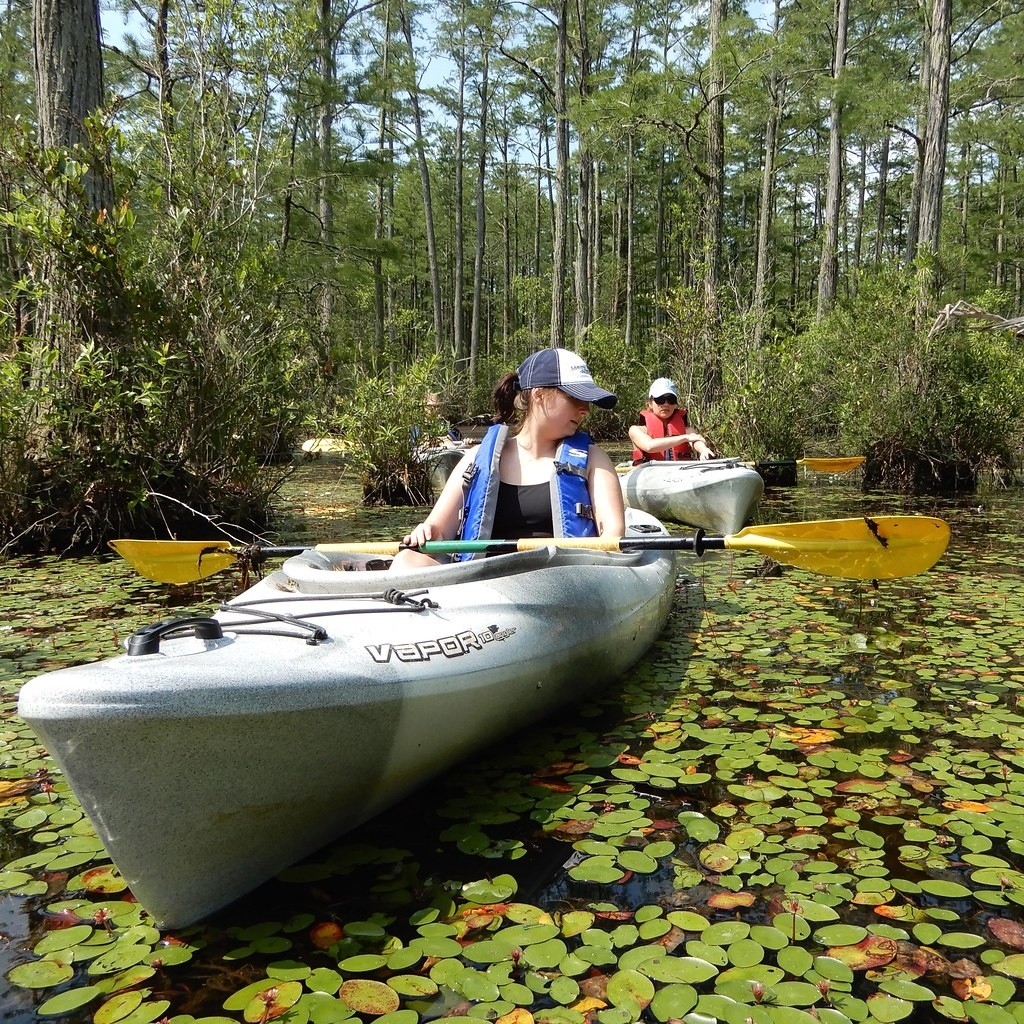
[
  {"x1": 103, "y1": 512, "x2": 953, "y2": 587},
  {"x1": 300, "y1": 436, "x2": 484, "y2": 456},
  {"x1": 614, "y1": 456, "x2": 868, "y2": 472}
]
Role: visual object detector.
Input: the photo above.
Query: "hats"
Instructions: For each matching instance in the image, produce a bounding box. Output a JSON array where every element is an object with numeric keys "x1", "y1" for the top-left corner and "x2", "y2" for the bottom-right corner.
[
  {"x1": 649, "y1": 378, "x2": 677, "y2": 399},
  {"x1": 513, "y1": 348, "x2": 617, "y2": 409}
]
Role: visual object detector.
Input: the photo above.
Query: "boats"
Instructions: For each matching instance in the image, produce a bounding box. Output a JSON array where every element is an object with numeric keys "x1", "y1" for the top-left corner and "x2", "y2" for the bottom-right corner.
[
  {"x1": 17, "y1": 507, "x2": 676, "y2": 929},
  {"x1": 615, "y1": 457, "x2": 764, "y2": 536},
  {"x1": 413, "y1": 446, "x2": 466, "y2": 490}
]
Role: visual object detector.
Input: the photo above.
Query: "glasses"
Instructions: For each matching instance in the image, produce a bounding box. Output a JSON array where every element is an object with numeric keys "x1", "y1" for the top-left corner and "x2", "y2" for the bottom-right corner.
[{"x1": 652, "y1": 394, "x2": 677, "y2": 405}]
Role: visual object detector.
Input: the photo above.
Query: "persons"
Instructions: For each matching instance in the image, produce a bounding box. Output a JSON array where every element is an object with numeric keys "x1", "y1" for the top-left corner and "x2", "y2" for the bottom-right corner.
[
  {"x1": 410, "y1": 393, "x2": 474, "y2": 447},
  {"x1": 402, "y1": 347, "x2": 626, "y2": 563},
  {"x1": 628, "y1": 377, "x2": 715, "y2": 466}
]
